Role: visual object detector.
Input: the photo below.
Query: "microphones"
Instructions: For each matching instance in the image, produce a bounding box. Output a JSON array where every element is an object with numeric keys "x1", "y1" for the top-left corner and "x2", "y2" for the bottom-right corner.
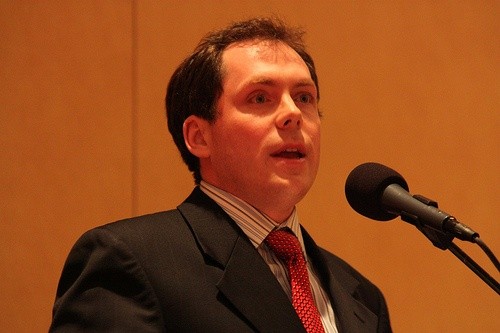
[{"x1": 344, "y1": 160, "x2": 480, "y2": 245}]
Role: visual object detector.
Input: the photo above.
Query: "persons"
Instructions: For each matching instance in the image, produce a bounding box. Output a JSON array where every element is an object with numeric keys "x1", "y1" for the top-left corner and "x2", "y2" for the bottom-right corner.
[{"x1": 47, "y1": 20, "x2": 393, "y2": 333}]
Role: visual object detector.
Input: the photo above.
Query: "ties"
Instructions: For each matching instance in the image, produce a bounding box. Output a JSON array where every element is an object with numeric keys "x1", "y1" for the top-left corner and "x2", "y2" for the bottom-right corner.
[{"x1": 269, "y1": 230, "x2": 326, "y2": 333}]
